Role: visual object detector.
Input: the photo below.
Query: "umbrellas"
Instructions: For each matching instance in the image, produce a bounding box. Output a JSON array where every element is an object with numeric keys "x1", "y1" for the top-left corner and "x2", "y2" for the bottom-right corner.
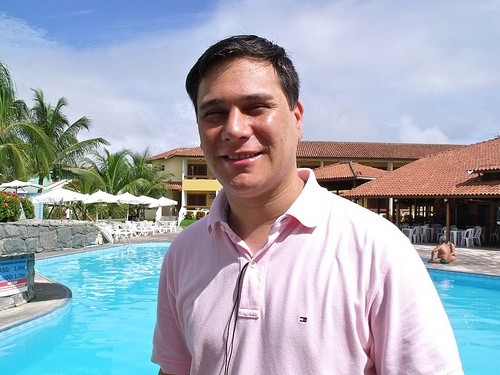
[
  {"x1": 0, "y1": 180, "x2": 44, "y2": 195},
  {"x1": 31, "y1": 189, "x2": 179, "y2": 222}
]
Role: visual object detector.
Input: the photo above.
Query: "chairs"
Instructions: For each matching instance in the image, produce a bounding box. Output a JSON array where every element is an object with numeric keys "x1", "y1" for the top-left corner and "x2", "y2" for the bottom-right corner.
[
  {"x1": 398, "y1": 221, "x2": 500, "y2": 248},
  {"x1": 95, "y1": 219, "x2": 178, "y2": 242}
]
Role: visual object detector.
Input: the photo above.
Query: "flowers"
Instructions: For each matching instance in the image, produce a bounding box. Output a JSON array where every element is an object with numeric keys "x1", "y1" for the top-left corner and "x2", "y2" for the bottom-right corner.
[{"x1": 3, "y1": 191, "x2": 19, "y2": 207}]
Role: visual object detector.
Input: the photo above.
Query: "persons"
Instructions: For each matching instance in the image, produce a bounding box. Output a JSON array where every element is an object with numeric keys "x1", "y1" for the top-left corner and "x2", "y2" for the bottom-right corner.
[
  {"x1": 64, "y1": 207, "x2": 72, "y2": 219},
  {"x1": 427, "y1": 236, "x2": 452, "y2": 264},
  {"x1": 151, "y1": 35, "x2": 469, "y2": 375},
  {"x1": 448, "y1": 241, "x2": 456, "y2": 261}
]
